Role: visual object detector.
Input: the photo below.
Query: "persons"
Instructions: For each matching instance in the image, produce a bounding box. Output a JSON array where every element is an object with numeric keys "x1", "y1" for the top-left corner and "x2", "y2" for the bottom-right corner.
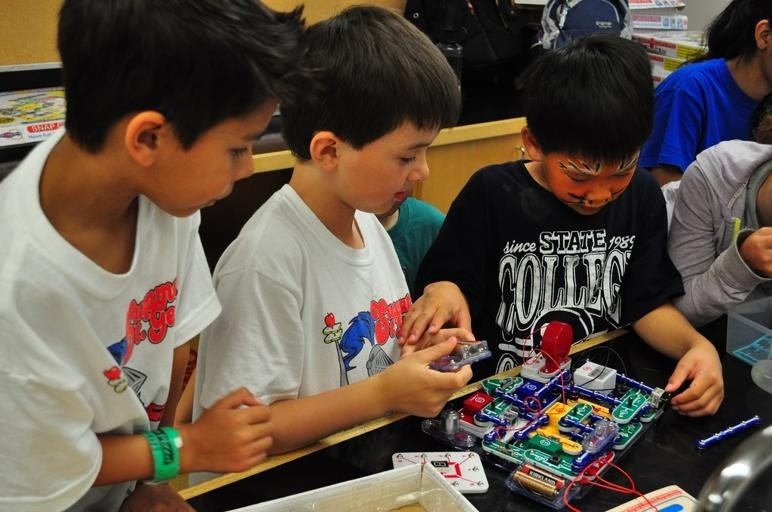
[
  {"x1": 640, "y1": 0, "x2": 772, "y2": 184},
  {"x1": 191, "y1": 4, "x2": 474, "y2": 492},
  {"x1": 0, "y1": 2, "x2": 304, "y2": 512},
  {"x1": 665, "y1": 136, "x2": 771, "y2": 336},
  {"x1": 398, "y1": 34, "x2": 727, "y2": 424},
  {"x1": 372, "y1": 194, "x2": 451, "y2": 296}
]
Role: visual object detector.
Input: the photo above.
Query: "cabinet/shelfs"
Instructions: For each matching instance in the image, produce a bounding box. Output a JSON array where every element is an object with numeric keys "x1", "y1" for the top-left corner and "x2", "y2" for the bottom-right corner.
[{"x1": 251, "y1": 117, "x2": 531, "y2": 217}]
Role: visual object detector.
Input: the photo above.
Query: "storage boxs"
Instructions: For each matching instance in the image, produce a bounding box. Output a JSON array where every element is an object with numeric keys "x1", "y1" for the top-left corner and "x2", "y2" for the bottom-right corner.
[{"x1": 726, "y1": 295, "x2": 771, "y2": 365}]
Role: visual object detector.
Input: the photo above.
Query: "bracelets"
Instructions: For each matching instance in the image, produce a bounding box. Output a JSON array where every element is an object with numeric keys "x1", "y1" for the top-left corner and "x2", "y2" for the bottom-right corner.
[{"x1": 144, "y1": 427, "x2": 182, "y2": 483}]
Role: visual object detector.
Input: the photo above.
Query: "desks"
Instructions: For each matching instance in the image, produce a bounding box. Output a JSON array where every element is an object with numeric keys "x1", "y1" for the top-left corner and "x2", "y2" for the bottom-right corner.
[{"x1": 174, "y1": 310, "x2": 771, "y2": 511}]
